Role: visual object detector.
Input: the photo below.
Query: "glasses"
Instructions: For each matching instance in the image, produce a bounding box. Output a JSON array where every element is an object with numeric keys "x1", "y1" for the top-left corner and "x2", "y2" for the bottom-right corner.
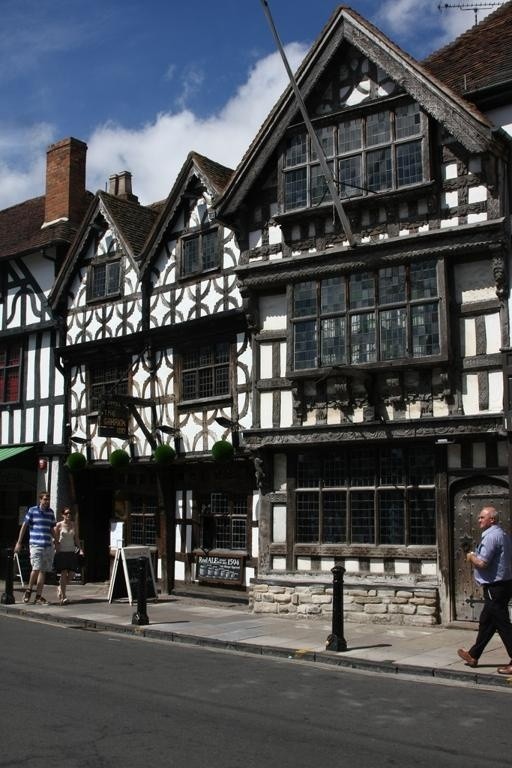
[
  {"x1": 63, "y1": 511, "x2": 71, "y2": 514},
  {"x1": 43, "y1": 498, "x2": 50, "y2": 501}
]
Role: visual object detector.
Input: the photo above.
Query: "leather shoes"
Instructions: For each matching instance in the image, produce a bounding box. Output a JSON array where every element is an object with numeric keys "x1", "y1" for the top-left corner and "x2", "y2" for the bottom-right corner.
[
  {"x1": 457, "y1": 648, "x2": 479, "y2": 667},
  {"x1": 497, "y1": 660, "x2": 512, "y2": 674}
]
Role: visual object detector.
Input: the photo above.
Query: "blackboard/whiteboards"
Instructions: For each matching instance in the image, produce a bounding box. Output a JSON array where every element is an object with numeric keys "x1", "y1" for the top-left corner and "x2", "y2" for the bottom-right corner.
[
  {"x1": 121, "y1": 546, "x2": 158, "y2": 600},
  {"x1": 194, "y1": 552, "x2": 244, "y2": 586},
  {"x1": 16, "y1": 552, "x2": 33, "y2": 582}
]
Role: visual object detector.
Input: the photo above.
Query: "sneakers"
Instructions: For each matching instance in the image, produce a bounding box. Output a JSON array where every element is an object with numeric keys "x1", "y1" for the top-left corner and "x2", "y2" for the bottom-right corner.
[
  {"x1": 33, "y1": 596, "x2": 51, "y2": 606},
  {"x1": 22, "y1": 588, "x2": 33, "y2": 602},
  {"x1": 57, "y1": 585, "x2": 69, "y2": 604}
]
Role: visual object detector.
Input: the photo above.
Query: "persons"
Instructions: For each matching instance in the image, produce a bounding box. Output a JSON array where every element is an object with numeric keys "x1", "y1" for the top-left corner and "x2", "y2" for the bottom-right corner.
[
  {"x1": 52, "y1": 504, "x2": 86, "y2": 605},
  {"x1": 456, "y1": 504, "x2": 511, "y2": 677},
  {"x1": 13, "y1": 491, "x2": 61, "y2": 605}
]
